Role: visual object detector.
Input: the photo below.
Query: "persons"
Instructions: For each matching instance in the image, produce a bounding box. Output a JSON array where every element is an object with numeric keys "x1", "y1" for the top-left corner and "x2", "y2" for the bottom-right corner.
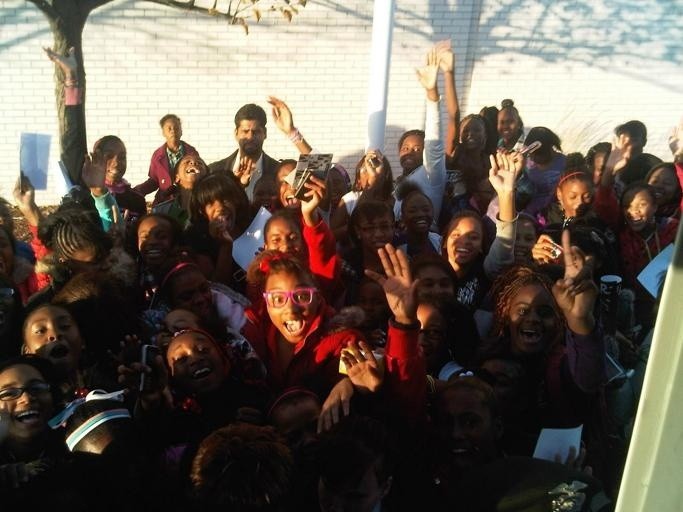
[{"x1": 0, "y1": 36, "x2": 683, "y2": 512}]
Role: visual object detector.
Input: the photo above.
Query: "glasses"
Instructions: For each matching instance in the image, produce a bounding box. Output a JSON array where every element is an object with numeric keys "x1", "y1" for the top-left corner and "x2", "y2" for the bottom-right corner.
[
  {"x1": 262, "y1": 287, "x2": 320, "y2": 309},
  {"x1": 0, "y1": 382, "x2": 52, "y2": 402}
]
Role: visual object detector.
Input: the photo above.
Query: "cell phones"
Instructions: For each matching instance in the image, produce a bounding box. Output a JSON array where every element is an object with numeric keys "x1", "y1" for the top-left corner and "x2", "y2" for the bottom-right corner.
[
  {"x1": 338, "y1": 349, "x2": 386, "y2": 379},
  {"x1": 139, "y1": 345, "x2": 163, "y2": 392},
  {"x1": 369, "y1": 149, "x2": 384, "y2": 168}
]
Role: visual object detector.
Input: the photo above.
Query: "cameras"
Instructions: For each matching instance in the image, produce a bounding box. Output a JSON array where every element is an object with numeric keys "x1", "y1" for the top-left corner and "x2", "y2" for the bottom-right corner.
[{"x1": 542, "y1": 240, "x2": 564, "y2": 265}]
